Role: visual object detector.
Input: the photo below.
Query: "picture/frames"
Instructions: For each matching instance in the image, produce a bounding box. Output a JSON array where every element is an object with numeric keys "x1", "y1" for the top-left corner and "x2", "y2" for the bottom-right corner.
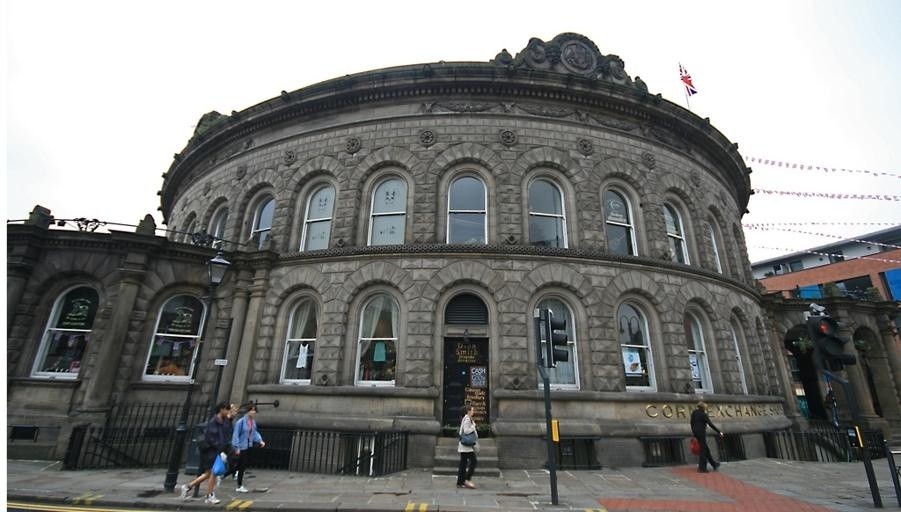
[{"x1": 470, "y1": 365, "x2": 488, "y2": 388}]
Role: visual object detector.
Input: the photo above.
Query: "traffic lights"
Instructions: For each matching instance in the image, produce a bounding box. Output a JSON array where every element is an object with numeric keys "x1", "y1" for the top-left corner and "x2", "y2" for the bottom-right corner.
[
  {"x1": 806, "y1": 314, "x2": 856, "y2": 373},
  {"x1": 544, "y1": 308, "x2": 568, "y2": 368}
]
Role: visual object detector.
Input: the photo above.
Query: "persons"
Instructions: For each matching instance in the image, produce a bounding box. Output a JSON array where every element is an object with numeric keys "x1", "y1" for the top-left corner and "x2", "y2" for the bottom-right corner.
[
  {"x1": 181, "y1": 401, "x2": 266, "y2": 504},
  {"x1": 691, "y1": 402, "x2": 723, "y2": 473},
  {"x1": 457, "y1": 405, "x2": 478, "y2": 489}
]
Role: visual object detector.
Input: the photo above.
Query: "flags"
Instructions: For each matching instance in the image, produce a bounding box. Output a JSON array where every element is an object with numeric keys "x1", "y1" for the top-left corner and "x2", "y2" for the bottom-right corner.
[{"x1": 679, "y1": 64, "x2": 697, "y2": 97}]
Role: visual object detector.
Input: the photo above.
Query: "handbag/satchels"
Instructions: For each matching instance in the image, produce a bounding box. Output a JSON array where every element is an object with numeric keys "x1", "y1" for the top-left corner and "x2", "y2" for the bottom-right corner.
[
  {"x1": 691, "y1": 438, "x2": 700, "y2": 454},
  {"x1": 196, "y1": 433, "x2": 211, "y2": 451},
  {"x1": 462, "y1": 432, "x2": 476, "y2": 445},
  {"x1": 212, "y1": 453, "x2": 228, "y2": 476}
]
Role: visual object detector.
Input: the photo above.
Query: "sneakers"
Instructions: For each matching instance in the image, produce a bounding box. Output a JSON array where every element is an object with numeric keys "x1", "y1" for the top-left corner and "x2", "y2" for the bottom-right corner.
[
  {"x1": 182, "y1": 483, "x2": 191, "y2": 501},
  {"x1": 235, "y1": 486, "x2": 248, "y2": 493},
  {"x1": 464, "y1": 481, "x2": 475, "y2": 488},
  {"x1": 698, "y1": 469, "x2": 709, "y2": 472},
  {"x1": 204, "y1": 493, "x2": 220, "y2": 504},
  {"x1": 457, "y1": 484, "x2": 466, "y2": 487},
  {"x1": 215, "y1": 476, "x2": 221, "y2": 488},
  {"x1": 714, "y1": 463, "x2": 719, "y2": 471}
]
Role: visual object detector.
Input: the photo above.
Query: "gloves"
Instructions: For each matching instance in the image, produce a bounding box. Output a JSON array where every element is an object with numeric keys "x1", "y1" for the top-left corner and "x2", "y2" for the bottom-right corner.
[{"x1": 220, "y1": 452, "x2": 227, "y2": 463}]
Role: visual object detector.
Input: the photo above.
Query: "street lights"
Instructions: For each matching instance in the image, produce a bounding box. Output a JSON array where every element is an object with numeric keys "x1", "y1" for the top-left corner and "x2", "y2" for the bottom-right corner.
[{"x1": 164, "y1": 252, "x2": 233, "y2": 494}]
